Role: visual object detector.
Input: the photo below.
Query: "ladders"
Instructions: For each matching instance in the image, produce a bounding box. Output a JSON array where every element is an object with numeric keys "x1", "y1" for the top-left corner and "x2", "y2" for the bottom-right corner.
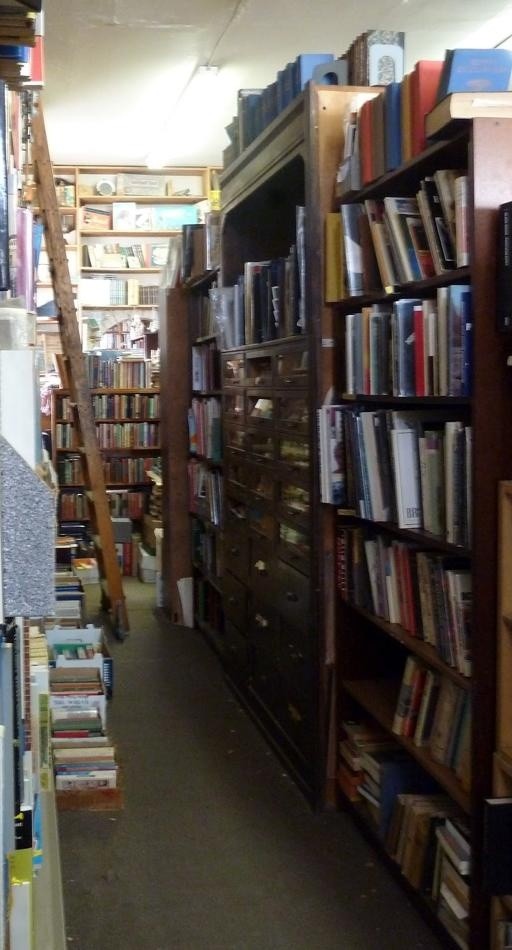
[{"x1": 24, "y1": 88, "x2": 132, "y2": 644}]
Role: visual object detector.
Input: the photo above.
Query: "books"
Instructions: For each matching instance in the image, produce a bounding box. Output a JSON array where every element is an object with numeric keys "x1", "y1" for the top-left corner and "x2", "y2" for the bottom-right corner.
[
  {"x1": 1, "y1": 587, "x2": 119, "y2": 809},
  {"x1": 1, "y1": 165, "x2": 190, "y2": 586},
  {"x1": 2, "y1": 796, "x2": 45, "y2": 950},
  {"x1": 189, "y1": 37, "x2": 511, "y2": 950},
  {"x1": 0, "y1": 0, "x2": 46, "y2": 166}
]
